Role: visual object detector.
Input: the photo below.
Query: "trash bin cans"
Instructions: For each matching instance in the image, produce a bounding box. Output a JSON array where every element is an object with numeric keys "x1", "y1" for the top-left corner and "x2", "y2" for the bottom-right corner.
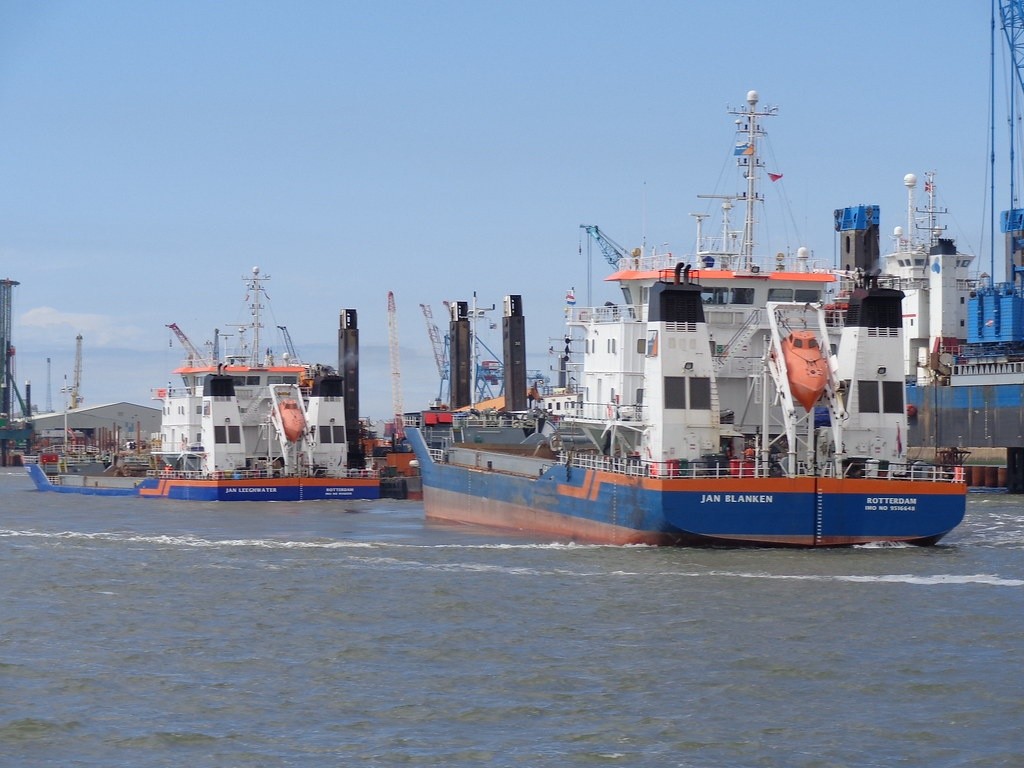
[
  {"x1": 730, "y1": 458, "x2": 756, "y2": 478},
  {"x1": 214, "y1": 470, "x2": 241, "y2": 479},
  {"x1": 703, "y1": 454, "x2": 727, "y2": 479},
  {"x1": 667, "y1": 458, "x2": 688, "y2": 479}
]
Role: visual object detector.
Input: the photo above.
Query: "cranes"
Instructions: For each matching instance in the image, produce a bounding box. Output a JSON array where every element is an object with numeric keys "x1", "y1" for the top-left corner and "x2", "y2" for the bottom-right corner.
[
  {"x1": 386, "y1": 289, "x2": 404, "y2": 452},
  {"x1": 60, "y1": 331, "x2": 84, "y2": 410},
  {"x1": 43, "y1": 357, "x2": 55, "y2": 413}
]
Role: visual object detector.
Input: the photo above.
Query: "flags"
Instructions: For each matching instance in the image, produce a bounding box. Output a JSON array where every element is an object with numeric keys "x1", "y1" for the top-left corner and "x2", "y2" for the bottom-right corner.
[
  {"x1": 767, "y1": 173, "x2": 783, "y2": 182},
  {"x1": 566, "y1": 295, "x2": 576, "y2": 306}
]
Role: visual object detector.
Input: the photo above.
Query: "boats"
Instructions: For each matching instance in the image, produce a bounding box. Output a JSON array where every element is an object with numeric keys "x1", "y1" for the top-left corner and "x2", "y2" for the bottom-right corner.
[
  {"x1": 402, "y1": 91, "x2": 969, "y2": 548},
  {"x1": 23, "y1": 261, "x2": 382, "y2": 502},
  {"x1": 369, "y1": 155, "x2": 1022, "y2": 492}
]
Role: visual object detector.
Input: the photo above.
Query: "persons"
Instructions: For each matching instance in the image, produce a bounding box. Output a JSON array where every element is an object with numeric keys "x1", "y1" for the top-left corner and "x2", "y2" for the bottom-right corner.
[
  {"x1": 165, "y1": 464, "x2": 174, "y2": 477},
  {"x1": 215, "y1": 465, "x2": 220, "y2": 475},
  {"x1": 741, "y1": 446, "x2": 754, "y2": 459}
]
